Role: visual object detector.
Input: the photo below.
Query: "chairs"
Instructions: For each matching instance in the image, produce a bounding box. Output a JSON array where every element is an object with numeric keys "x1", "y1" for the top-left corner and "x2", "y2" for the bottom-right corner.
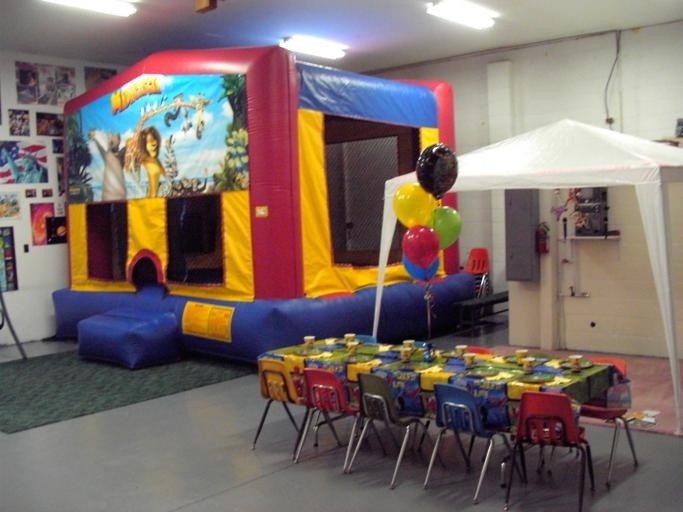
[{"x1": 461, "y1": 249, "x2": 490, "y2": 317}]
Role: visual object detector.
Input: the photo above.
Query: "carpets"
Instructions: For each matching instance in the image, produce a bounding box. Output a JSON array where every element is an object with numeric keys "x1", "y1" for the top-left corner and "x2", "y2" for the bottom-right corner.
[{"x1": 1, "y1": 346, "x2": 259, "y2": 433}]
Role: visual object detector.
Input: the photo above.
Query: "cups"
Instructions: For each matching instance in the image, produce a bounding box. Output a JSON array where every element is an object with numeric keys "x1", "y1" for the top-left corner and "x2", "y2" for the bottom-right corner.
[
  {"x1": 515, "y1": 349, "x2": 528, "y2": 366},
  {"x1": 522, "y1": 358, "x2": 535, "y2": 374},
  {"x1": 347, "y1": 342, "x2": 358, "y2": 356},
  {"x1": 344, "y1": 332, "x2": 356, "y2": 350},
  {"x1": 435, "y1": 350, "x2": 443, "y2": 362},
  {"x1": 303, "y1": 335, "x2": 317, "y2": 353},
  {"x1": 462, "y1": 354, "x2": 476, "y2": 369},
  {"x1": 567, "y1": 355, "x2": 583, "y2": 373},
  {"x1": 399, "y1": 348, "x2": 412, "y2": 364},
  {"x1": 455, "y1": 346, "x2": 469, "y2": 360},
  {"x1": 403, "y1": 340, "x2": 415, "y2": 355}
]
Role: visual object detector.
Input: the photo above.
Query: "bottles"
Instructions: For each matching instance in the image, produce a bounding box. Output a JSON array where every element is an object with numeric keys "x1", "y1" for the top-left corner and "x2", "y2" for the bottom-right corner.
[{"x1": 423, "y1": 339, "x2": 433, "y2": 362}]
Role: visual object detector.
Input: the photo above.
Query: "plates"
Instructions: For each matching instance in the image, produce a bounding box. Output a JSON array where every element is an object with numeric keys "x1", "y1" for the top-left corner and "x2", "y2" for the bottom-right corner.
[
  {"x1": 456, "y1": 367, "x2": 501, "y2": 378},
  {"x1": 293, "y1": 348, "x2": 324, "y2": 356},
  {"x1": 515, "y1": 372, "x2": 556, "y2": 384},
  {"x1": 341, "y1": 355, "x2": 376, "y2": 364},
  {"x1": 558, "y1": 360, "x2": 595, "y2": 370}
]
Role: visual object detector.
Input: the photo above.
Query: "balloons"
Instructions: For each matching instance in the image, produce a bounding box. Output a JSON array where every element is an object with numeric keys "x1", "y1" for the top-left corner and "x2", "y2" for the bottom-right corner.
[
  {"x1": 410, "y1": 181, "x2": 441, "y2": 212},
  {"x1": 399, "y1": 252, "x2": 439, "y2": 281},
  {"x1": 428, "y1": 207, "x2": 462, "y2": 249},
  {"x1": 416, "y1": 144, "x2": 459, "y2": 200},
  {"x1": 401, "y1": 225, "x2": 439, "y2": 271},
  {"x1": 392, "y1": 186, "x2": 428, "y2": 228}
]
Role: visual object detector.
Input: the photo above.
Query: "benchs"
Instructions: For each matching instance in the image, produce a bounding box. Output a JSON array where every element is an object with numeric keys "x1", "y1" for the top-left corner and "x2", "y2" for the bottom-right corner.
[{"x1": 453, "y1": 290, "x2": 508, "y2": 337}]
